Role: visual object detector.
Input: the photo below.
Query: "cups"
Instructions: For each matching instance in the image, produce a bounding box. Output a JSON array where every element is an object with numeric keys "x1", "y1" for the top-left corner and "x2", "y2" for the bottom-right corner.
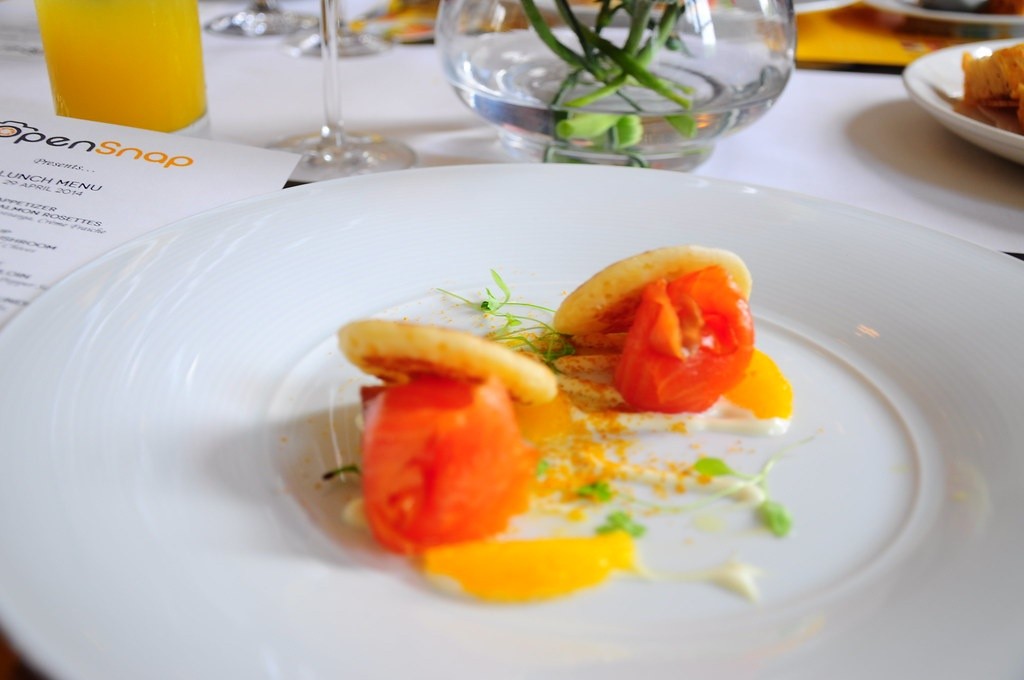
[{"x1": 34, "y1": 0, "x2": 210, "y2": 147}]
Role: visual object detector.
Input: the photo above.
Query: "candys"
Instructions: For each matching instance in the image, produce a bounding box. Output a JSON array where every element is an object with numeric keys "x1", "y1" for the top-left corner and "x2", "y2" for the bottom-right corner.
[{"x1": 357, "y1": 370, "x2": 532, "y2": 554}]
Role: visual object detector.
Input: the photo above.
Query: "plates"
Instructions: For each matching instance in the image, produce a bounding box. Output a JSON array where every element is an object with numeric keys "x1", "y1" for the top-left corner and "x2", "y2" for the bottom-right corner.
[
  {"x1": 0, "y1": 164, "x2": 1024, "y2": 679},
  {"x1": 865, "y1": 0, "x2": 1024, "y2": 26},
  {"x1": 902, "y1": 38, "x2": 1024, "y2": 165}
]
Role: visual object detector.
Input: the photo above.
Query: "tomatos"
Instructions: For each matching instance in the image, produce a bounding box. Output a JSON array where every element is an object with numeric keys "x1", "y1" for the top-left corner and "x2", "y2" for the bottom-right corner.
[{"x1": 614, "y1": 263, "x2": 755, "y2": 414}]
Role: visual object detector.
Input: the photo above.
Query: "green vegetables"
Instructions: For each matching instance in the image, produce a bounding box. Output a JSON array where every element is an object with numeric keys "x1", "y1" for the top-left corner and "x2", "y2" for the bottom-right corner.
[{"x1": 435, "y1": 268, "x2": 575, "y2": 375}]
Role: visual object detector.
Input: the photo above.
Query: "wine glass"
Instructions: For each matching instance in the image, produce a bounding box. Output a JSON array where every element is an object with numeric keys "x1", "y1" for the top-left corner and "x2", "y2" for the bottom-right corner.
[
  {"x1": 265, "y1": 0, "x2": 419, "y2": 182},
  {"x1": 202, "y1": 0, "x2": 323, "y2": 44}
]
